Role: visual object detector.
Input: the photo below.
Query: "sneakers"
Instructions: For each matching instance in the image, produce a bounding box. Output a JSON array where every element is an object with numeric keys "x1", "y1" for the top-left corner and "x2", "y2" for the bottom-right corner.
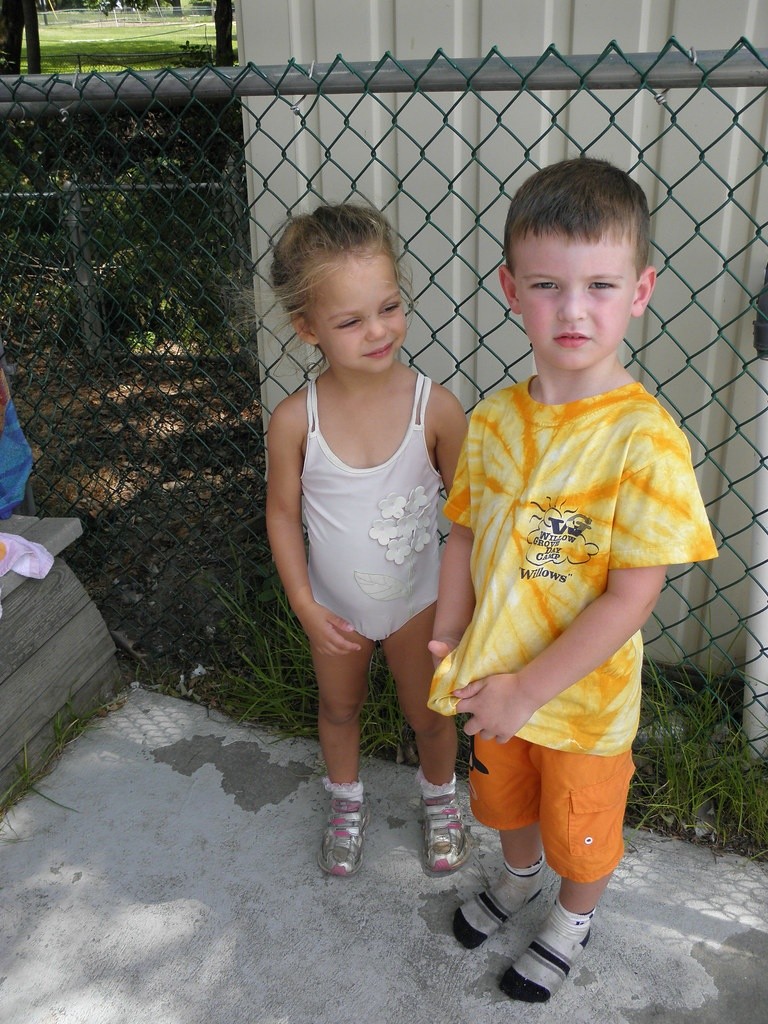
[
  {"x1": 420, "y1": 793, "x2": 470, "y2": 872},
  {"x1": 317, "y1": 794, "x2": 371, "y2": 876}
]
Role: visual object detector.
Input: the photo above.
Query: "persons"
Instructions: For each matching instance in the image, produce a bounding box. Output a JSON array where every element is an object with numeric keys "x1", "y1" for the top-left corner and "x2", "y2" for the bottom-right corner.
[
  {"x1": 429, "y1": 158, "x2": 717, "y2": 1002},
  {"x1": 266, "y1": 205, "x2": 467, "y2": 875}
]
